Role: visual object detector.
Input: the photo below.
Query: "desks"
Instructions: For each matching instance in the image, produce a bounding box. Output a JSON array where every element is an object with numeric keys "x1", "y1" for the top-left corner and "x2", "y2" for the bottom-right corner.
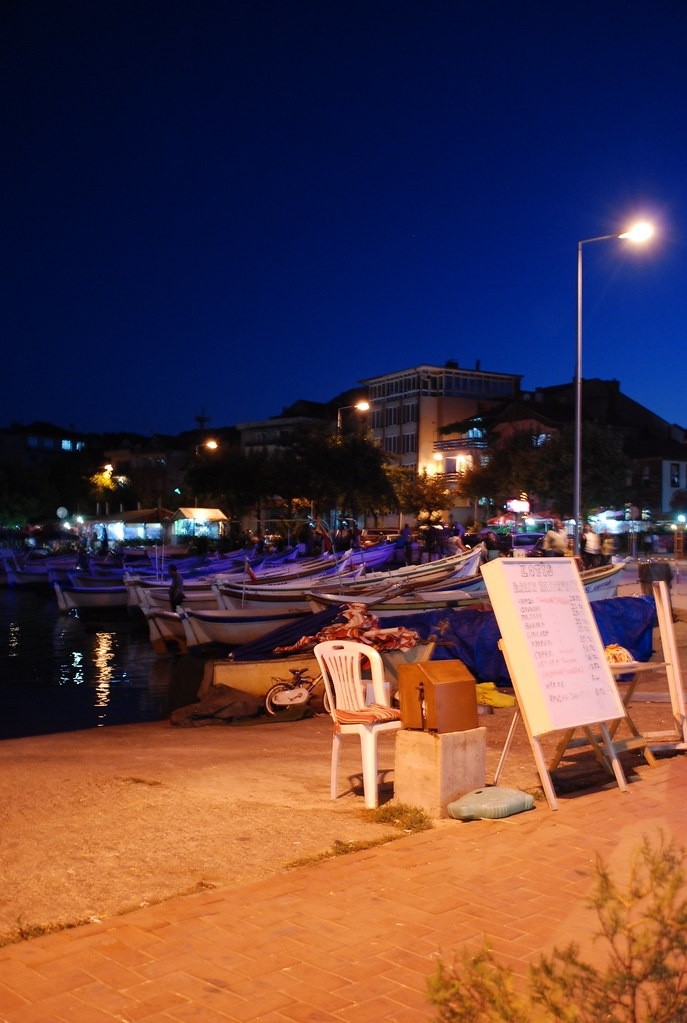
[{"x1": 548, "y1": 662, "x2": 671, "y2": 792}]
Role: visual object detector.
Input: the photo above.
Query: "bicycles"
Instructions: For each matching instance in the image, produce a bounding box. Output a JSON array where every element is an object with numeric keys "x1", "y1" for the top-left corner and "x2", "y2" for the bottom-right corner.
[{"x1": 263, "y1": 667, "x2": 329, "y2": 715}]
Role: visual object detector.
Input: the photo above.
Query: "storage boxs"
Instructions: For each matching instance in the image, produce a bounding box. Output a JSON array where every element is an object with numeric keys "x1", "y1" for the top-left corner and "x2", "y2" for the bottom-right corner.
[{"x1": 397, "y1": 660, "x2": 479, "y2": 734}]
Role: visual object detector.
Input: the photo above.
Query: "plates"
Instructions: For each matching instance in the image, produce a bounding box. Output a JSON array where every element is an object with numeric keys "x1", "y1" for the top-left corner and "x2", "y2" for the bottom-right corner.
[{"x1": 610, "y1": 661, "x2": 639, "y2": 668}]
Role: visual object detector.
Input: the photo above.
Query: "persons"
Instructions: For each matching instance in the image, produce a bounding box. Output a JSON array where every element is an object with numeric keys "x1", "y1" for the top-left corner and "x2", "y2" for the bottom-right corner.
[
  {"x1": 75, "y1": 525, "x2": 108, "y2": 570},
  {"x1": 542, "y1": 521, "x2": 568, "y2": 557},
  {"x1": 353, "y1": 526, "x2": 358, "y2": 542},
  {"x1": 581, "y1": 524, "x2": 602, "y2": 566},
  {"x1": 168, "y1": 564, "x2": 183, "y2": 613},
  {"x1": 600, "y1": 528, "x2": 619, "y2": 565},
  {"x1": 481, "y1": 532, "x2": 500, "y2": 549},
  {"x1": 418, "y1": 521, "x2": 438, "y2": 563},
  {"x1": 444, "y1": 529, "x2": 468, "y2": 555},
  {"x1": 399, "y1": 524, "x2": 412, "y2": 536},
  {"x1": 295, "y1": 538, "x2": 305, "y2": 557},
  {"x1": 255, "y1": 536, "x2": 264, "y2": 551}
]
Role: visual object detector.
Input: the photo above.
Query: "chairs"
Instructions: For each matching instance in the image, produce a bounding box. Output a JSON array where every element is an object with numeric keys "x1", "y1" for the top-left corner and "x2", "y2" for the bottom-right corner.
[{"x1": 314, "y1": 640, "x2": 401, "y2": 810}]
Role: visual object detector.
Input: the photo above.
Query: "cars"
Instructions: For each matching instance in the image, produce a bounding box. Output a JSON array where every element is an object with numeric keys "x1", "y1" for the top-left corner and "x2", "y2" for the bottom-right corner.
[
  {"x1": 360, "y1": 528, "x2": 400, "y2": 545},
  {"x1": 505, "y1": 531, "x2": 545, "y2": 555}
]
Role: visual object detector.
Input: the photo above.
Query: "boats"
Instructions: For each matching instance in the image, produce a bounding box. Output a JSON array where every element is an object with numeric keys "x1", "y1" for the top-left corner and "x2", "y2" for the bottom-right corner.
[{"x1": 0, "y1": 540, "x2": 654, "y2": 712}]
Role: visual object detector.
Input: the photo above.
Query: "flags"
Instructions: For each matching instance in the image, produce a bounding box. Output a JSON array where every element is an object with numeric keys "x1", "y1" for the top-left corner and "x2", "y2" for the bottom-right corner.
[{"x1": 246, "y1": 564, "x2": 256, "y2": 581}]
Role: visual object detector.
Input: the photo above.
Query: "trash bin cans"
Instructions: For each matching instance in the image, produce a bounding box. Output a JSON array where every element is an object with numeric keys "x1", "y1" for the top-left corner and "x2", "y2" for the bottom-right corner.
[{"x1": 639, "y1": 563, "x2": 673, "y2": 596}]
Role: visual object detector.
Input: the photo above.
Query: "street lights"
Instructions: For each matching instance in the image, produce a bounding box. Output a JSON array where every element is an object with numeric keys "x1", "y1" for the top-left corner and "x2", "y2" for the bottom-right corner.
[
  {"x1": 336, "y1": 399, "x2": 371, "y2": 443},
  {"x1": 195, "y1": 439, "x2": 216, "y2": 508},
  {"x1": 572, "y1": 222, "x2": 654, "y2": 556}
]
